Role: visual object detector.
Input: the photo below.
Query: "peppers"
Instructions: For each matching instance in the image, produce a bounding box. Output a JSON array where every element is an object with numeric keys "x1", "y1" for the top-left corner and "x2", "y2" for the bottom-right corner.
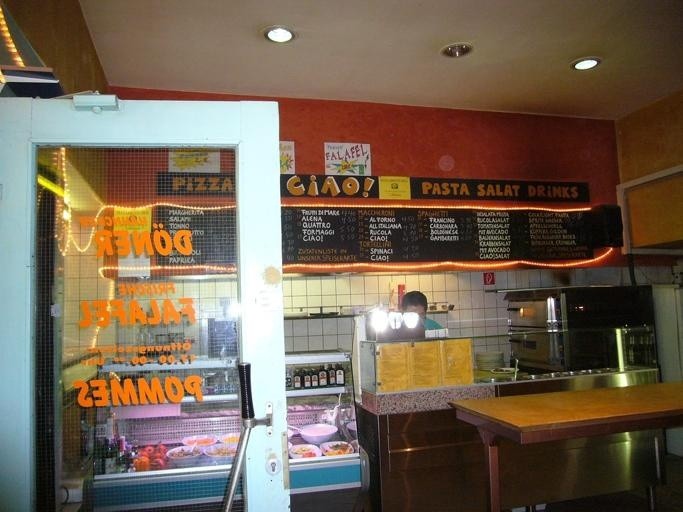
[{"x1": 133, "y1": 443, "x2": 168, "y2": 471}]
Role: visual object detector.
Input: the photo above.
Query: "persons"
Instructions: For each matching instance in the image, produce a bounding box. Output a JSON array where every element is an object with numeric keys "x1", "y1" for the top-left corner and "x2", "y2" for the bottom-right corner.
[{"x1": 401, "y1": 291, "x2": 446, "y2": 329}]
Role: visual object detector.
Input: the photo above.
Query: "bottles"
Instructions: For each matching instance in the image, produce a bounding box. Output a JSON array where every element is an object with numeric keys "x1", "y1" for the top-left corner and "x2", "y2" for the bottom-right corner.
[
  {"x1": 293, "y1": 362, "x2": 346, "y2": 389},
  {"x1": 105, "y1": 436, "x2": 139, "y2": 473}
]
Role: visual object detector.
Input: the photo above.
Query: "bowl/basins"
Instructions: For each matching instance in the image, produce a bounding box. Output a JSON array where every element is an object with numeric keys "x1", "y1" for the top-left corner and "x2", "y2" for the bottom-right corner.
[
  {"x1": 181, "y1": 434, "x2": 218, "y2": 449},
  {"x1": 298, "y1": 423, "x2": 339, "y2": 445},
  {"x1": 218, "y1": 432, "x2": 241, "y2": 444},
  {"x1": 310, "y1": 312, "x2": 339, "y2": 316},
  {"x1": 284, "y1": 312, "x2": 308, "y2": 318},
  {"x1": 165, "y1": 445, "x2": 204, "y2": 467},
  {"x1": 203, "y1": 443, "x2": 239, "y2": 463}
]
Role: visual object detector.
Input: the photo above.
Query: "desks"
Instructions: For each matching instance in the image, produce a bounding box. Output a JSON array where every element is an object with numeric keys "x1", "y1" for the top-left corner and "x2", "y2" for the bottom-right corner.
[{"x1": 447, "y1": 380, "x2": 682, "y2": 512}]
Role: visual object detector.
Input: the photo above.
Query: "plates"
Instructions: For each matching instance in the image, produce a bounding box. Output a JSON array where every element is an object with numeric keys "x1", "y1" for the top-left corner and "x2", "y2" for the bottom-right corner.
[
  {"x1": 289, "y1": 444, "x2": 323, "y2": 459},
  {"x1": 491, "y1": 367, "x2": 519, "y2": 374},
  {"x1": 319, "y1": 440, "x2": 355, "y2": 456},
  {"x1": 475, "y1": 350, "x2": 507, "y2": 372}
]
